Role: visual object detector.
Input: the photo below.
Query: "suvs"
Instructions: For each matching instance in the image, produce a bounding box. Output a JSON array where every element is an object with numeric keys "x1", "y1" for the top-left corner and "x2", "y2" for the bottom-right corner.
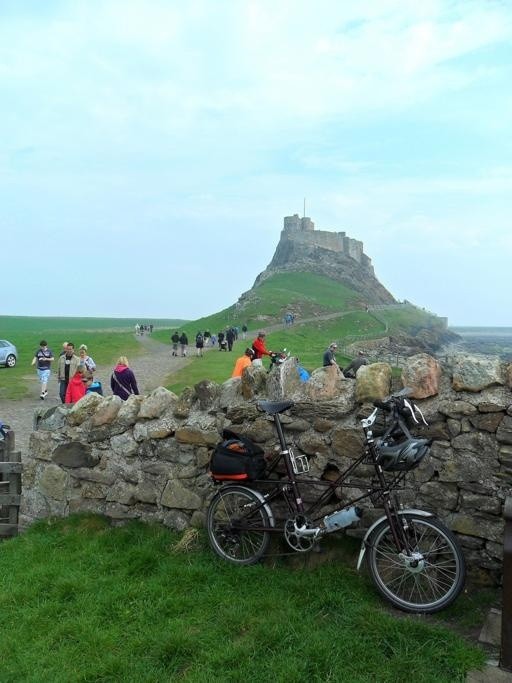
[{"x1": 0, "y1": 339, "x2": 17, "y2": 368}]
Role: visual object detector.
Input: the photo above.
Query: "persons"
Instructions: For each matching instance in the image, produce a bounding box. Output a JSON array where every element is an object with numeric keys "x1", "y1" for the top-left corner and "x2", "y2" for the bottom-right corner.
[
  {"x1": 81, "y1": 371, "x2": 104, "y2": 396},
  {"x1": 31, "y1": 339, "x2": 55, "y2": 400},
  {"x1": 65, "y1": 364, "x2": 87, "y2": 404},
  {"x1": 109, "y1": 355, "x2": 140, "y2": 400},
  {"x1": 295, "y1": 357, "x2": 310, "y2": 383},
  {"x1": 283, "y1": 312, "x2": 294, "y2": 326},
  {"x1": 134, "y1": 322, "x2": 152, "y2": 337},
  {"x1": 322, "y1": 342, "x2": 337, "y2": 366},
  {"x1": 60, "y1": 341, "x2": 70, "y2": 356},
  {"x1": 77, "y1": 343, "x2": 97, "y2": 382},
  {"x1": 232, "y1": 347, "x2": 255, "y2": 378},
  {"x1": 170, "y1": 322, "x2": 248, "y2": 358},
  {"x1": 342, "y1": 350, "x2": 370, "y2": 377},
  {"x1": 57, "y1": 343, "x2": 80, "y2": 402},
  {"x1": 251, "y1": 331, "x2": 276, "y2": 368}
]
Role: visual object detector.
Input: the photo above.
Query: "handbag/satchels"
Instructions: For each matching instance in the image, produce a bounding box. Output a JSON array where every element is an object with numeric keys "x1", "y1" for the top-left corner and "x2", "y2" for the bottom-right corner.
[{"x1": 208, "y1": 428, "x2": 267, "y2": 481}]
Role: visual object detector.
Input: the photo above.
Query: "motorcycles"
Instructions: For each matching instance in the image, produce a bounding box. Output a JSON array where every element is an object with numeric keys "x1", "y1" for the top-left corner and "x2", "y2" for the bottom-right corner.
[{"x1": 268, "y1": 346, "x2": 291, "y2": 372}]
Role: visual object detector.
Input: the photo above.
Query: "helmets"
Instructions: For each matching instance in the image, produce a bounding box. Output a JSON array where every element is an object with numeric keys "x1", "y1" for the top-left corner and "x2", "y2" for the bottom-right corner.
[
  {"x1": 376, "y1": 438, "x2": 433, "y2": 471},
  {"x1": 246, "y1": 348, "x2": 255, "y2": 356}
]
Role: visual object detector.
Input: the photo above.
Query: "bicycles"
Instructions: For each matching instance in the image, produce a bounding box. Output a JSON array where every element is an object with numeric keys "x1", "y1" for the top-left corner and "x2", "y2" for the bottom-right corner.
[{"x1": 205, "y1": 394, "x2": 465, "y2": 614}]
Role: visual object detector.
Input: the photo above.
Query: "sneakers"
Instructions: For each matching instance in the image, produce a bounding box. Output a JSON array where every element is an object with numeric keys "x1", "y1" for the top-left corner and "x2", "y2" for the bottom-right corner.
[{"x1": 39, "y1": 389, "x2": 48, "y2": 401}]
